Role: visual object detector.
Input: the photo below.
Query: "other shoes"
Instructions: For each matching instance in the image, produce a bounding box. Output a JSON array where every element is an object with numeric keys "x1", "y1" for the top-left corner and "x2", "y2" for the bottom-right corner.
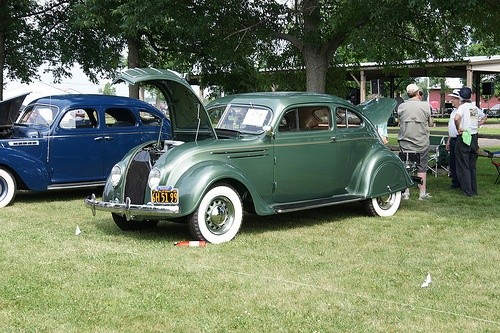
[{"x1": 402, "y1": 192, "x2": 432, "y2": 200}]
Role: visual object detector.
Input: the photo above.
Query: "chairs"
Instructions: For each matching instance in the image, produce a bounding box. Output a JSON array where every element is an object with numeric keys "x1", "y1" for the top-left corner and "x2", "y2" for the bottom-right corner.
[
  {"x1": 283, "y1": 110, "x2": 319, "y2": 132},
  {"x1": 426, "y1": 143, "x2": 453, "y2": 177}
]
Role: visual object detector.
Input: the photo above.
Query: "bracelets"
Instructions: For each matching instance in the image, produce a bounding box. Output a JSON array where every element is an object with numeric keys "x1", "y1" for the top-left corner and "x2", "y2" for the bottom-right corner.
[{"x1": 447, "y1": 141, "x2": 451, "y2": 142}]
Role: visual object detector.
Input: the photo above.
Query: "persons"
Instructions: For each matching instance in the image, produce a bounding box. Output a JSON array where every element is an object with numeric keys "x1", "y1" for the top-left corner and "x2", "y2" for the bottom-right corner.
[
  {"x1": 395, "y1": 83, "x2": 433, "y2": 201},
  {"x1": 453, "y1": 86, "x2": 487, "y2": 198},
  {"x1": 393, "y1": 92, "x2": 404, "y2": 112},
  {"x1": 445, "y1": 90, "x2": 463, "y2": 188}
]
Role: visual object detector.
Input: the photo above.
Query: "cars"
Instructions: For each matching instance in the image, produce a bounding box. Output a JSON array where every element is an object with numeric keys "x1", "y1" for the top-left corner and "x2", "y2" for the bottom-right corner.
[
  {"x1": 443, "y1": 100, "x2": 500, "y2": 118},
  {"x1": 0, "y1": 93, "x2": 173, "y2": 209},
  {"x1": 84, "y1": 68, "x2": 425, "y2": 245}
]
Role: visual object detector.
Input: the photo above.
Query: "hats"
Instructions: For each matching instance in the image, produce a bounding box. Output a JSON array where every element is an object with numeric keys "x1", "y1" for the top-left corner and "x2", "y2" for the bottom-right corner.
[
  {"x1": 448, "y1": 88, "x2": 463, "y2": 100},
  {"x1": 406, "y1": 84, "x2": 420, "y2": 93},
  {"x1": 458, "y1": 88, "x2": 471, "y2": 99}
]
